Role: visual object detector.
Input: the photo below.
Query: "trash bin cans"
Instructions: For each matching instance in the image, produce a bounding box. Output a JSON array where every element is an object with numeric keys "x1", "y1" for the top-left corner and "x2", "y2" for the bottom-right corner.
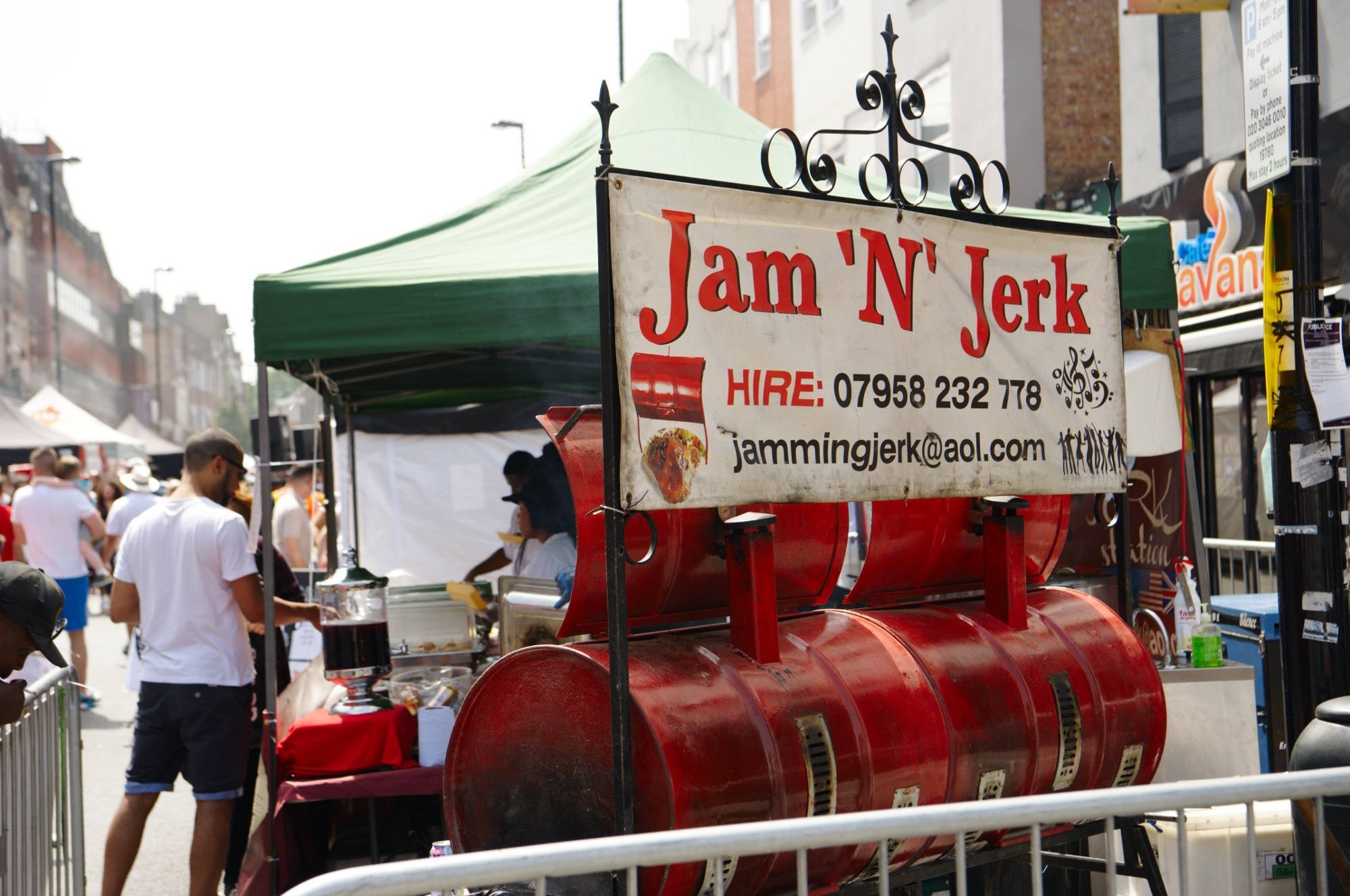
[{"x1": 1290, "y1": 695, "x2": 1350, "y2": 896}]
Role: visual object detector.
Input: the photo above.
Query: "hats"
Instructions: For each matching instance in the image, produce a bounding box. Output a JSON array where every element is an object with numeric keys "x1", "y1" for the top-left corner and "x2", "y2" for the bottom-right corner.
[
  {"x1": 0, "y1": 561, "x2": 66, "y2": 668},
  {"x1": 120, "y1": 465, "x2": 162, "y2": 493},
  {"x1": 503, "y1": 475, "x2": 559, "y2": 531}
]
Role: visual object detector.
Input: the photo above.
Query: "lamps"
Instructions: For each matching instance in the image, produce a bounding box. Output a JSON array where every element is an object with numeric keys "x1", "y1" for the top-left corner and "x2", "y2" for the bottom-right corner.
[{"x1": 314, "y1": 546, "x2": 395, "y2": 715}]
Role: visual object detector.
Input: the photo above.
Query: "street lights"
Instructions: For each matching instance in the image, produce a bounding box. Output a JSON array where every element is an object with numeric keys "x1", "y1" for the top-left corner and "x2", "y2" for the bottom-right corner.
[
  {"x1": 153, "y1": 265, "x2": 177, "y2": 434},
  {"x1": 45, "y1": 153, "x2": 84, "y2": 393},
  {"x1": 490, "y1": 119, "x2": 526, "y2": 172}
]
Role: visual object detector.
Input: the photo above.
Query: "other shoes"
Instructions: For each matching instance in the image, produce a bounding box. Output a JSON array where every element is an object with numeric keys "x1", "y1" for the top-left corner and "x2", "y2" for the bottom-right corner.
[
  {"x1": 78, "y1": 691, "x2": 97, "y2": 708},
  {"x1": 92, "y1": 572, "x2": 115, "y2": 586}
]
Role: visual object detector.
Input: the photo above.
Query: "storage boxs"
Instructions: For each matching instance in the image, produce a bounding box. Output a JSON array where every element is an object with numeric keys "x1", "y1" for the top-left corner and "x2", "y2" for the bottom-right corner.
[{"x1": 387, "y1": 580, "x2": 495, "y2": 674}]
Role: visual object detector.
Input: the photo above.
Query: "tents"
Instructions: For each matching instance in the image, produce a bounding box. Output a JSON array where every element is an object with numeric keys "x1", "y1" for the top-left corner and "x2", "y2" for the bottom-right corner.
[
  {"x1": 253, "y1": 53, "x2": 1213, "y2": 721},
  {"x1": 0, "y1": 384, "x2": 185, "y2": 472}
]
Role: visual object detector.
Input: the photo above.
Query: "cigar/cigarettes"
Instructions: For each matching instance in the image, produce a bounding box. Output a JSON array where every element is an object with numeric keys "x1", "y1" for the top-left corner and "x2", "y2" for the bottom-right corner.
[{"x1": 23, "y1": 689, "x2": 36, "y2": 695}]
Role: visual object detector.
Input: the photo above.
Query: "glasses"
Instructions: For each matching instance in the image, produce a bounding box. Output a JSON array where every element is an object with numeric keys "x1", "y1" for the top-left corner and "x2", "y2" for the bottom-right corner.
[
  {"x1": 211, "y1": 451, "x2": 247, "y2": 480},
  {"x1": 4, "y1": 596, "x2": 68, "y2": 643}
]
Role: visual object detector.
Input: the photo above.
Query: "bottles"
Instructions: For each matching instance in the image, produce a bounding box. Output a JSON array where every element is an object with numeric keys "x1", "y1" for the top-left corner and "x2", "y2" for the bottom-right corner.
[{"x1": 1173, "y1": 556, "x2": 1201, "y2": 656}]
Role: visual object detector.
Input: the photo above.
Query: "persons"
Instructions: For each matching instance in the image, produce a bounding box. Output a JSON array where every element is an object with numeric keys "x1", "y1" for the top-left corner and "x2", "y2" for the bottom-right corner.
[
  {"x1": 0, "y1": 561, "x2": 68, "y2": 727},
  {"x1": 223, "y1": 485, "x2": 306, "y2": 896},
  {"x1": 102, "y1": 428, "x2": 341, "y2": 896},
  {"x1": 272, "y1": 465, "x2": 313, "y2": 566},
  {"x1": 10, "y1": 447, "x2": 112, "y2": 710},
  {"x1": 463, "y1": 450, "x2": 577, "y2": 623},
  {"x1": 98, "y1": 457, "x2": 163, "y2": 654}
]
